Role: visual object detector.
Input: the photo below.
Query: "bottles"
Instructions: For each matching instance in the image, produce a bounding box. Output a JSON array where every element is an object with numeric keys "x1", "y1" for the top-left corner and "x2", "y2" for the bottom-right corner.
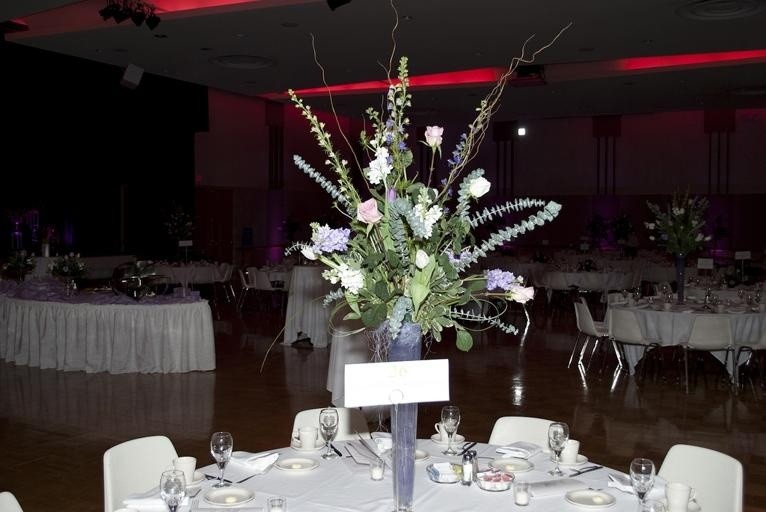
[
  {"x1": 467, "y1": 450, "x2": 478, "y2": 481},
  {"x1": 461, "y1": 454, "x2": 473, "y2": 486}
]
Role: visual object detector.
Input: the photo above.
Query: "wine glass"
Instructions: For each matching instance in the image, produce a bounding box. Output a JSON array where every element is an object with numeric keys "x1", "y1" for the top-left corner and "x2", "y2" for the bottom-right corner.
[
  {"x1": 633, "y1": 287, "x2": 642, "y2": 304},
  {"x1": 319, "y1": 408, "x2": 339, "y2": 460},
  {"x1": 746, "y1": 293, "x2": 754, "y2": 305},
  {"x1": 160, "y1": 470, "x2": 186, "y2": 512},
  {"x1": 439, "y1": 405, "x2": 461, "y2": 456},
  {"x1": 621, "y1": 289, "x2": 630, "y2": 300},
  {"x1": 547, "y1": 422, "x2": 569, "y2": 478},
  {"x1": 665, "y1": 292, "x2": 674, "y2": 303},
  {"x1": 630, "y1": 458, "x2": 656, "y2": 504},
  {"x1": 637, "y1": 499, "x2": 665, "y2": 512}
]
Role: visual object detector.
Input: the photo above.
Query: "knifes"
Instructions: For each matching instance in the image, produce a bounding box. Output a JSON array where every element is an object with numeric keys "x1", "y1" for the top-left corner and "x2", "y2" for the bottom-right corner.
[
  {"x1": 458, "y1": 441, "x2": 478, "y2": 455},
  {"x1": 570, "y1": 466, "x2": 603, "y2": 478},
  {"x1": 205, "y1": 474, "x2": 232, "y2": 483},
  {"x1": 325, "y1": 442, "x2": 342, "y2": 457}
]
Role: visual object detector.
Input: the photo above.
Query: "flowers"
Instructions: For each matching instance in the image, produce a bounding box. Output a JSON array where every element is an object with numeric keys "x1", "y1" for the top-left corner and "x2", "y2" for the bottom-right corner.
[
  {"x1": 258, "y1": 0, "x2": 573, "y2": 374},
  {"x1": 643, "y1": 184, "x2": 711, "y2": 252}
]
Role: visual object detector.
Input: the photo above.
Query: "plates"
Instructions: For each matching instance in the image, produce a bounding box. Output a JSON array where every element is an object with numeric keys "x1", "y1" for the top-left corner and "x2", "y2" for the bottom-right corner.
[
  {"x1": 565, "y1": 489, "x2": 616, "y2": 509},
  {"x1": 551, "y1": 454, "x2": 588, "y2": 467},
  {"x1": 386, "y1": 448, "x2": 431, "y2": 463},
  {"x1": 291, "y1": 440, "x2": 325, "y2": 451},
  {"x1": 425, "y1": 463, "x2": 463, "y2": 484},
  {"x1": 203, "y1": 486, "x2": 255, "y2": 506},
  {"x1": 473, "y1": 471, "x2": 515, "y2": 491},
  {"x1": 487, "y1": 457, "x2": 535, "y2": 473},
  {"x1": 274, "y1": 457, "x2": 321, "y2": 471},
  {"x1": 430, "y1": 434, "x2": 465, "y2": 445},
  {"x1": 186, "y1": 471, "x2": 206, "y2": 487}
]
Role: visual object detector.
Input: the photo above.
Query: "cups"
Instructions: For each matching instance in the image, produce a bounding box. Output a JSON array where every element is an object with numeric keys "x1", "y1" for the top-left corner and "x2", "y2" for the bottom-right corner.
[
  {"x1": 369, "y1": 458, "x2": 385, "y2": 481},
  {"x1": 174, "y1": 456, "x2": 197, "y2": 484},
  {"x1": 665, "y1": 482, "x2": 696, "y2": 512},
  {"x1": 513, "y1": 482, "x2": 530, "y2": 507},
  {"x1": 267, "y1": 495, "x2": 288, "y2": 512},
  {"x1": 435, "y1": 421, "x2": 456, "y2": 442},
  {"x1": 209, "y1": 432, "x2": 233, "y2": 488},
  {"x1": 561, "y1": 440, "x2": 580, "y2": 463},
  {"x1": 298, "y1": 425, "x2": 317, "y2": 448}
]
